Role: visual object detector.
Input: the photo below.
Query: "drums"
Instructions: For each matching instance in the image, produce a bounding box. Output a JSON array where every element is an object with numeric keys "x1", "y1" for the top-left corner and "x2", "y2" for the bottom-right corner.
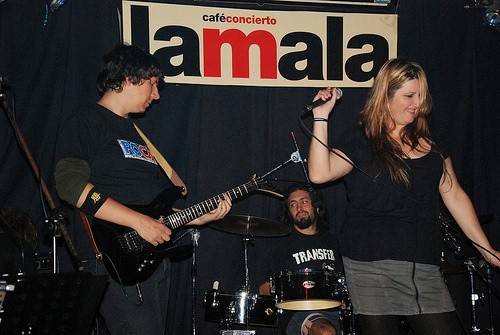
[
  {"x1": 272, "y1": 267, "x2": 346, "y2": 311},
  {"x1": 203, "y1": 290, "x2": 279, "y2": 328}
]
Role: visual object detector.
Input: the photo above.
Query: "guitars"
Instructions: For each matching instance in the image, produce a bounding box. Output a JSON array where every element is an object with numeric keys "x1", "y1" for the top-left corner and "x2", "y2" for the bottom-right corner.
[{"x1": 92, "y1": 173, "x2": 285, "y2": 286}]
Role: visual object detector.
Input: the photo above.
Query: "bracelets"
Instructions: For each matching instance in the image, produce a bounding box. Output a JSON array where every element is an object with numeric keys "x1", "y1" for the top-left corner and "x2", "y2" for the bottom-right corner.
[
  {"x1": 489, "y1": 250, "x2": 498, "y2": 264},
  {"x1": 313, "y1": 118, "x2": 328, "y2": 121}
]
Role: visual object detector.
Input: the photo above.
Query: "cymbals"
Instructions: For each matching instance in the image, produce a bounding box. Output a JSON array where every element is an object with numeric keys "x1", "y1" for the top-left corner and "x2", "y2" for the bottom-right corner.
[{"x1": 206, "y1": 214, "x2": 293, "y2": 237}]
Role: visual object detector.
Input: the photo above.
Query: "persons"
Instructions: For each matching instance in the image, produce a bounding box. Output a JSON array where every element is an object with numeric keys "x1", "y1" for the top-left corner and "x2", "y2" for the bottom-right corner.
[
  {"x1": 53, "y1": 43, "x2": 232, "y2": 335},
  {"x1": 258, "y1": 182, "x2": 351, "y2": 296},
  {"x1": 307, "y1": 58, "x2": 500, "y2": 335}
]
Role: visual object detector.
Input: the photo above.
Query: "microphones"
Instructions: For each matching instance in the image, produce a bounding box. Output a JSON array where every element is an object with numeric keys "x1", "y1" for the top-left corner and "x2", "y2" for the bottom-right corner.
[{"x1": 299, "y1": 88, "x2": 342, "y2": 115}]
